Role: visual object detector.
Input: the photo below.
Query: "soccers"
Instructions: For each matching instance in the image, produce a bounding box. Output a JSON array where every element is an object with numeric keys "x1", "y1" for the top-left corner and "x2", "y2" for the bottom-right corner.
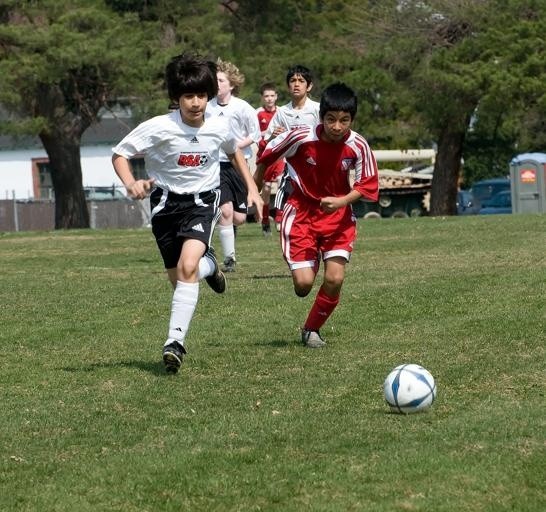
[{"x1": 385, "y1": 363, "x2": 436, "y2": 414}]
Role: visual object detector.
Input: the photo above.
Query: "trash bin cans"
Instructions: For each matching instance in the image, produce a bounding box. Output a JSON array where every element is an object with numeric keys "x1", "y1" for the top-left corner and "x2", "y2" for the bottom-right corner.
[{"x1": 509, "y1": 152, "x2": 546, "y2": 215}]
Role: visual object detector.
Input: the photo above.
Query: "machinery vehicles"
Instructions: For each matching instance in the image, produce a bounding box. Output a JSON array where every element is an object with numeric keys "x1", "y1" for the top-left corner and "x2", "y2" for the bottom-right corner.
[{"x1": 347, "y1": 143, "x2": 436, "y2": 223}]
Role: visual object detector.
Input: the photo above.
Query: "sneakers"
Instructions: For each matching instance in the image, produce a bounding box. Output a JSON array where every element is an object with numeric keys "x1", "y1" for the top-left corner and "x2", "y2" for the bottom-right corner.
[
  {"x1": 262, "y1": 220, "x2": 272, "y2": 237},
  {"x1": 202, "y1": 247, "x2": 227, "y2": 293},
  {"x1": 162, "y1": 341, "x2": 186, "y2": 375},
  {"x1": 276, "y1": 222, "x2": 281, "y2": 232},
  {"x1": 302, "y1": 328, "x2": 327, "y2": 348},
  {"x1": 221, "y1": 257, "x2": 236, "y2": 273}
]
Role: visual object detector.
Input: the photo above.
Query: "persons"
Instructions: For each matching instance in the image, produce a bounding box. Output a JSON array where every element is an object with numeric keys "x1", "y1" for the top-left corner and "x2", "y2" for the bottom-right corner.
[
  {"x1": 253, "y1": 81, "x2": 379, "y2": 349},
  {"x1": 201, "y1": 62, "x2": 265, "y2": 271},
  {"x1": 111, "y1": 63, "x2": 266, "y2": 374},
  {"x1": 260, "y1": 64, "x2": 324, "y2": 230},
  {"x1": 457, "y1": 182, "x2": 484, "y2": 215},
  {"x1": 254, "y1": 84, "x2": 286, "y2": 230}
]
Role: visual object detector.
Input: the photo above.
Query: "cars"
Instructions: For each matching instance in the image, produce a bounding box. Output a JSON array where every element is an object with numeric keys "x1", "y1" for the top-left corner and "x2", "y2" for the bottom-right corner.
[{"x1": 455, "y1": 174, "x2": 512, "y2": 217}]
